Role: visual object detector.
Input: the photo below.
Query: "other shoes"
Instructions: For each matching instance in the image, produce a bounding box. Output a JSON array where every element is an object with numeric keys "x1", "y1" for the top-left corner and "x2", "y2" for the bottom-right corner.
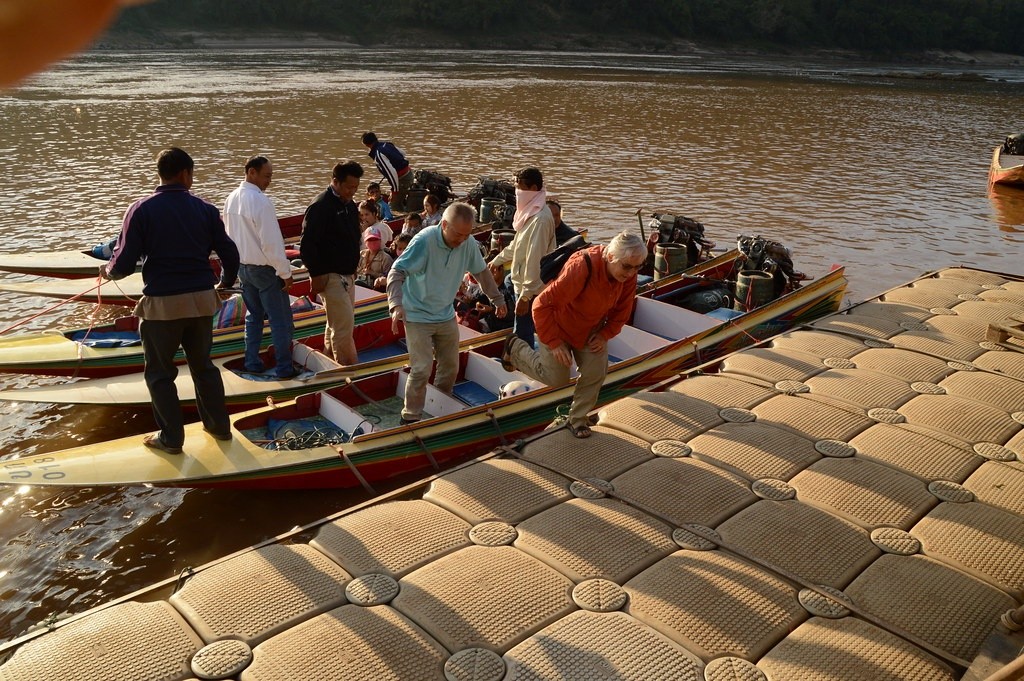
[
  {"x1": 400, "y1": 416, "x2": 419, "y2": 425},
  {"x1": 279, "y1": 367, "x2": 303, "y2": 380}
]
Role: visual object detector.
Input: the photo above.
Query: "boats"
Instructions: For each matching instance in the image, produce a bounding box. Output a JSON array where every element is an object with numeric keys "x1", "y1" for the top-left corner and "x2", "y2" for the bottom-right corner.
[
  {"x1": 0, "y1": 170, "x2": 850, "y2": 497},
  {"x1": 988, "y1": 132, "x2": 1024, "y2": 190}
]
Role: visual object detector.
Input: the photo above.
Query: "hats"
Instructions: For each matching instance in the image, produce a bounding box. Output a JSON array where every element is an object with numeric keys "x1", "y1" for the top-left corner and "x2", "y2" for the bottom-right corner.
[{"x1": 364, "y1": 226, "x2": 382, "y2": 240}]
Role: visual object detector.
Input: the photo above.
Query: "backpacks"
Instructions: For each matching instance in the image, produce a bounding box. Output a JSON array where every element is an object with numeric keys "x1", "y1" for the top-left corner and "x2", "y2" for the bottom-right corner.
[{"x1": 540, "y1": 235, "x2": 592, "y2": 293}]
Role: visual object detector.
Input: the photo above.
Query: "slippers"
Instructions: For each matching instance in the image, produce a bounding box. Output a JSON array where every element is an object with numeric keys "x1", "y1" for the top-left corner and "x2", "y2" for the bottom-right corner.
[
  {"x1": 203, "y1": 427, "x2": 232, "y2": 440},
  {"x1": 143, "y1": 432, "x2": 182, "y2": 454}
]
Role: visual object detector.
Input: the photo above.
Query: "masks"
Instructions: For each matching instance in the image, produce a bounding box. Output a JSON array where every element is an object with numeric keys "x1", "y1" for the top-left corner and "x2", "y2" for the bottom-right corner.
[{"x1": 366, "y1": 241, "x2": 380, "y2": 250}]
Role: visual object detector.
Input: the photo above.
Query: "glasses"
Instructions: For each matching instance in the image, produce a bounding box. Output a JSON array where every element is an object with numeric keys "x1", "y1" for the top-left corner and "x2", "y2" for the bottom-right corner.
[
  {"x1": 620, "y1": 261, "x2": 643, "y2": 271},
  {"x1": 512, "y1": 176, "x2": 528, "y2": 185}
]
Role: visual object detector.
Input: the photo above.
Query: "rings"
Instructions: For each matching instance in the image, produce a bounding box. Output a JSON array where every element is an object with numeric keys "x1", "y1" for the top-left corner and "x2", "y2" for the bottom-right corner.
[{"x1": 288, "y1": 286, "x2": 291, "y2": 288}]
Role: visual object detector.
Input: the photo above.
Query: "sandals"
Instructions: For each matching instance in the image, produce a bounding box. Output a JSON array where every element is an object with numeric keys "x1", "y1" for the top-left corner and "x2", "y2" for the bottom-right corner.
[
  {"x1": 501, "y1": 334, "x2": 518, "y2": 372},
  {"x1": 566, "y1": 421, "x2": 592, "y2": 438}
]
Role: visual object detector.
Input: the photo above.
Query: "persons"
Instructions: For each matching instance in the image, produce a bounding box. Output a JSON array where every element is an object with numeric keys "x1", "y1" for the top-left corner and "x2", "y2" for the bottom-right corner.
[
  {"x1": 99, "y1": 148, "x2": 240, "y2": 454},
  {"x1": 361, "y1": 132, "x2": 413, "y2": 209},
  {"x1": 358, "y1": 183, "x2": 585, "y2": 333},
  {"x1": 299, "y1": 160, "x2": 364, "y2": 365},
  {"x1": 502, "y1": 230, "x2": 648, "y2": 438},
  {"x1": 488, "y1": 168, "x2": 557, "y2": 350},
  {"x1": 1, "y1": 0, "x2": 153, "y2": 99},
  {"x1": 223, "y1": 156, "x2": 298, "y2": 379},
  {"x1": 385, "y1": 203, "x2": 507, "y2": 425}
]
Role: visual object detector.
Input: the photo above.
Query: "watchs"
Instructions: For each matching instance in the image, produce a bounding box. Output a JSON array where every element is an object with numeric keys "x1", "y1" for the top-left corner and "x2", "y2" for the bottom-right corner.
[{"x1": 520, "y1": 296, "x2": 530, "y2": 303}]
